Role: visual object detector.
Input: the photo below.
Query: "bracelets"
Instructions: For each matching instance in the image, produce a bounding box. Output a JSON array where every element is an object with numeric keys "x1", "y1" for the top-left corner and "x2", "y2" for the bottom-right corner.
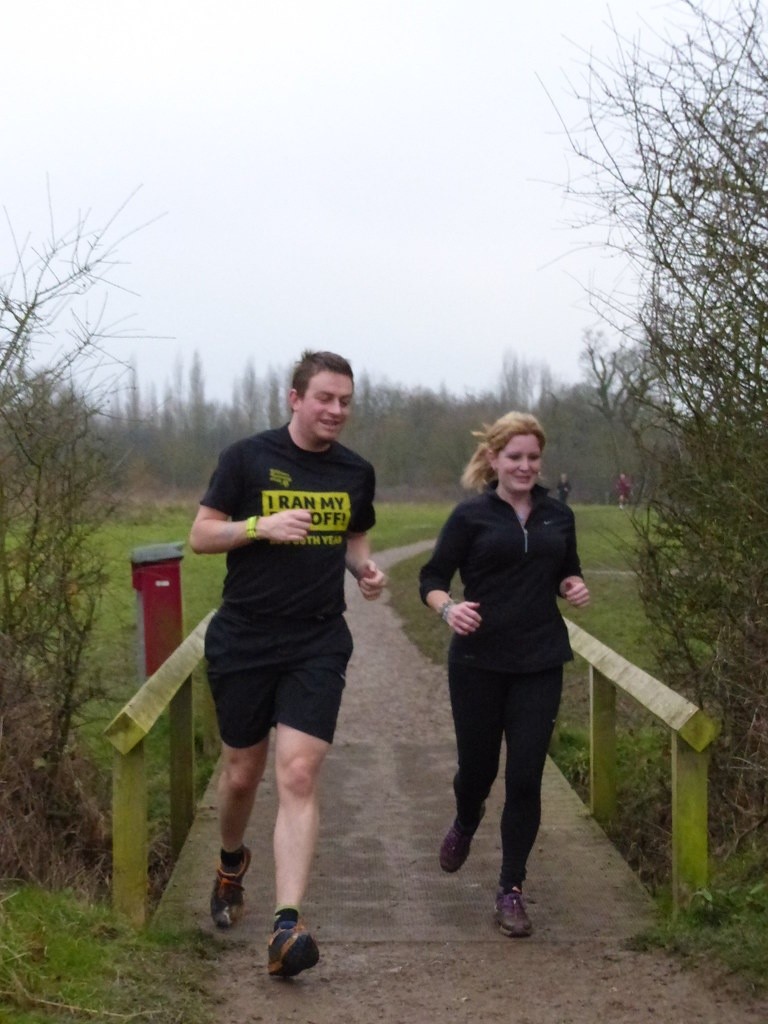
[
  {"x1": 247, "y1": 516, "x2": 259, "y2": 541},
  {"x1": 438, "y1": 601, "x2": 451, "y2": 622}
]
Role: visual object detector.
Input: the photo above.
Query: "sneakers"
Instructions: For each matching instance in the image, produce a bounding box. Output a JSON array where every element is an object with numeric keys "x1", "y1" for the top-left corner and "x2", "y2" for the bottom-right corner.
[
  {"x1": 440, "y1": 799, "x2": 488, "y2": 873},
  {"x1": 210, "y1": 846, "x2": 251, "y2": 927},
  {"x1": 494, "y1": 887, "x2": 531, "y2": 939},
  {"x1": 266, "y1": 922, "x2": 318, "y2": 978}
]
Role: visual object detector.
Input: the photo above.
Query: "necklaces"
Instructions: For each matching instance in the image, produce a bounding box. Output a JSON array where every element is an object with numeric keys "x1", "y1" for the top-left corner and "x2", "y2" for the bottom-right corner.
[{"x1": 518, "y1": 514, "x2": 523, "y2": 521}]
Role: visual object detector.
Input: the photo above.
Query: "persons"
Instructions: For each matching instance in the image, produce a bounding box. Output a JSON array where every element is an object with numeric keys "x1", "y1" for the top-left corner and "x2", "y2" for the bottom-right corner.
[
  {"x1": 191, "y1": 351, "x2": 385, "y2": 979},
  {"x1": 556, "y1": 474, "x2": 571, "y2": 504},
  {"x1": 615, "y1": 474, "x2": 632, "y2": 511},
  {"x1": 419, "y1": 411, "x2": 590, "y2": 937}
]
